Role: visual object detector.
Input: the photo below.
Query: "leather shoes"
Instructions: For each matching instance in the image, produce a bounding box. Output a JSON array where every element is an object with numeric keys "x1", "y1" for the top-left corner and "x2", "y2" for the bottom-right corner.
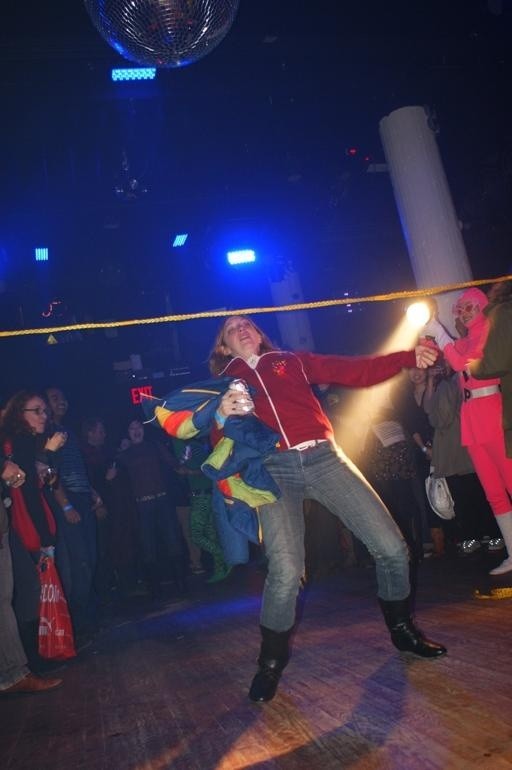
[{"x1": 0, "y1": 671, "x2": 63, "y2": 694}]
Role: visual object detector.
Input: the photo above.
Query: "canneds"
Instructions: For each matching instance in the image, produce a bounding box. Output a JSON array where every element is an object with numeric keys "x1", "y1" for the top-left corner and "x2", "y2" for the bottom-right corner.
[{"x1": 228, "y1": 378, "x2": 256, "y2": 415}]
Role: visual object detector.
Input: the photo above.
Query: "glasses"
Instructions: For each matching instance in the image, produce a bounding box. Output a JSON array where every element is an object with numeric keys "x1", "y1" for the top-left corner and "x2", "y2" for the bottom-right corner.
[
  {"x1": 456, "y1": 302, "x2": 477, "y2": 315},
  {"x1": 24, "y1": 408, "x2": 48, "y2": 414}
]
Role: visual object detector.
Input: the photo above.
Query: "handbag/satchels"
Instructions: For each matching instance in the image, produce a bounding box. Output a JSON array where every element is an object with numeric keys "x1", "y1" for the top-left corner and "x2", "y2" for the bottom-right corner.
[{"x1": 38, "y1": 556, "x2": 76, "y2": 658}]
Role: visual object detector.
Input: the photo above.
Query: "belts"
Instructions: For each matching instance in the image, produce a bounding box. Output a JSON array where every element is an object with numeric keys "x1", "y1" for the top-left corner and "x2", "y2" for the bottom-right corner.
[{"x1": 463, "y1": 385, "x2": 501, "y2": 402}]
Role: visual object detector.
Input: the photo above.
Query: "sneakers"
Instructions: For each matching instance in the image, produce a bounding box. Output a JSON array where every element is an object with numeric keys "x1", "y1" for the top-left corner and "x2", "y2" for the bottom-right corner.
[{"x1": 462, "y1": 538, "x2": 503, "y2": 553}]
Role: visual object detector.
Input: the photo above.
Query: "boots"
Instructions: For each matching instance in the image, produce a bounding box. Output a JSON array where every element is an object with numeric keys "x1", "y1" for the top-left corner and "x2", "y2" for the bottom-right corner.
[
  {"x1": 380, "y1": 597, "x2": 447, "y2": 660},
  {"x1": 249, "y1": 624, "x2": 294, "y2": 704},
  {"x1": 490, "y1": 511, "x2": 512, "y2": 575}
]
